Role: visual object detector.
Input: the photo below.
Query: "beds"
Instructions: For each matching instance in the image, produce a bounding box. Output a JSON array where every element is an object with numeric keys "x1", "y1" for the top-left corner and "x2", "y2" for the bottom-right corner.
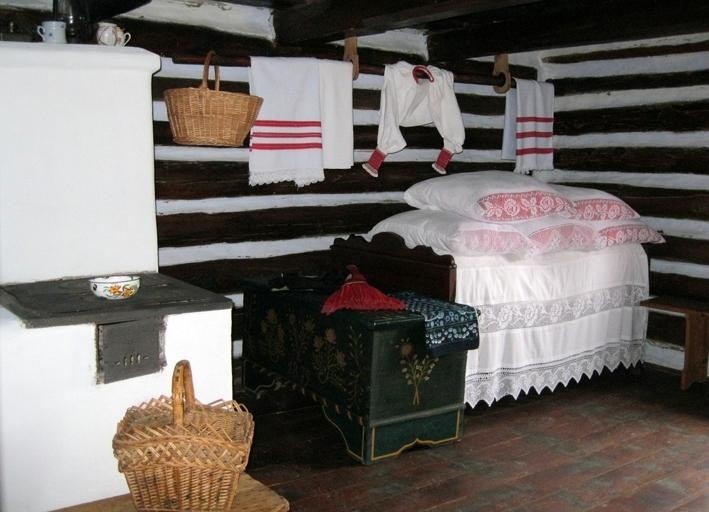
[{"x1": 331, "y1": 231, "x2": 651, "y2": 412}]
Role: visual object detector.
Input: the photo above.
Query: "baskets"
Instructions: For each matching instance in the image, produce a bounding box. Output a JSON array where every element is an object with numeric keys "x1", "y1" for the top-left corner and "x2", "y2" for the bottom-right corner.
[
  {"x1": 114, "y1": 360, "x2": 255, "y2": 511},
  {"x1": 163, "y1": 52, "x2": 263, "y2": 147}
]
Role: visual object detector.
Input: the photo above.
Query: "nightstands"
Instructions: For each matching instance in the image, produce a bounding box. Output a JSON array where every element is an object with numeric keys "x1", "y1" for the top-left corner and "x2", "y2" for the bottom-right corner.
[{"x1": 639, "y1": 294, "x2": 707, "y2": 391}]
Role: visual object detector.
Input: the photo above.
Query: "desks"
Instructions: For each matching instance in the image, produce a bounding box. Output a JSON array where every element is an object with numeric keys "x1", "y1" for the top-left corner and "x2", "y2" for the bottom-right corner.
[
  {"x1": 58, "y1": 470, "x2": 289, "y2": 511},
  {"x1": 242, "y1": 271, "x2": 481, "y2": 467}
]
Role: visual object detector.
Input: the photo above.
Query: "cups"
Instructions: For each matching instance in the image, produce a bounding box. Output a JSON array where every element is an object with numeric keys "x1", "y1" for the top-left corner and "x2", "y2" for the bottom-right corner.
[
  {"x1": 36, "y1": 20, "x2": 67, "y2": 44},
  {"x1": 116, "y1": 27, "x2": 131, "y2": 45}
]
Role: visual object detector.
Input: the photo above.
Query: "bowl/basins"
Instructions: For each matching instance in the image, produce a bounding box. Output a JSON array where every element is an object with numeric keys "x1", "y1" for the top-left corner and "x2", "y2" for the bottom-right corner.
[{"x1": 90, "y1": 275, "x2": 141, "y2": 300}]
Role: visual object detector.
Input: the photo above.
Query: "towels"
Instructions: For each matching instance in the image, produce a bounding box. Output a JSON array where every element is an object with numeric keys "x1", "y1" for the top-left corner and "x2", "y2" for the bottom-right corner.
[
  {"x1": 501, "y1": 77, "x2": 555, "y2": 173},
  {"x1": 248, "y1": 55, "x2": 354, "y2": 185}
]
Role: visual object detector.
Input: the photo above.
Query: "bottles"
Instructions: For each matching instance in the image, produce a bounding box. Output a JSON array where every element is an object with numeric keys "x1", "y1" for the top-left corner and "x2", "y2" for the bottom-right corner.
[{"x1": 95, "y1": 21, "x2": 116, "y2": 45}]
